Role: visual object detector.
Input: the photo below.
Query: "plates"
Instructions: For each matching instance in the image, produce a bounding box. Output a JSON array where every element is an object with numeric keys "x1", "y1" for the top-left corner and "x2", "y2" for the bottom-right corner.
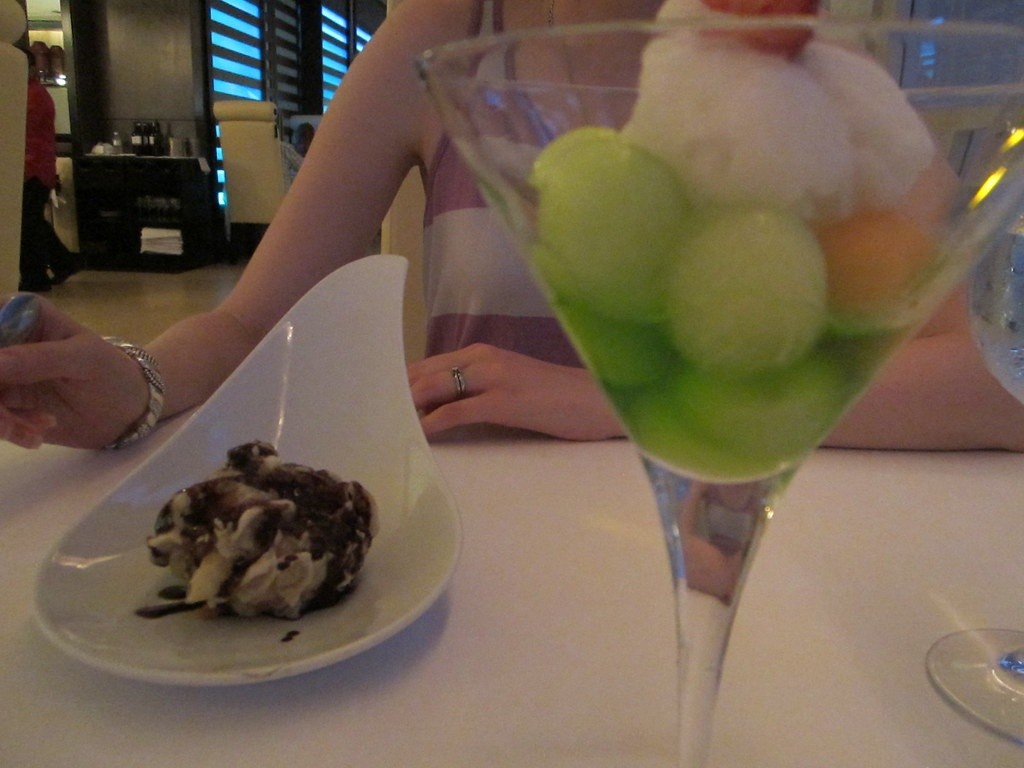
[{"x1": 29, "y1": 251, "x2": 464, "y2": 687}]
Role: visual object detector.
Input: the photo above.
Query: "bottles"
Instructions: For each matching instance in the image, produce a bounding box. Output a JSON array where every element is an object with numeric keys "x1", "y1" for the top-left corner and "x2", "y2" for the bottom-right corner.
[
  {"x1": 131, "y1": 119, "x2": 172, "y2": 156},
  {"x1": 29, "y1": 40, "x2": 65, "y2": 84},
  {"x1": 113, "y1": 132, "x2": 123, "y2": 154}
]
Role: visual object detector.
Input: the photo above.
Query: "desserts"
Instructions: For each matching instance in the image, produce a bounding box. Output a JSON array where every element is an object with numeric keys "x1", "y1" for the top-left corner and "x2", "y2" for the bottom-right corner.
[{"x1": 145, "y1": 440, "x2": 379, "y2": 622}]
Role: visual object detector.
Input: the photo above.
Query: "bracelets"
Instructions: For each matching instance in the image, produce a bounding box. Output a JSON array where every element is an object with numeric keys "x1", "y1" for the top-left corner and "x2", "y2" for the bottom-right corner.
[{"x1": 101, "y1": 334, "x2": 165, "y2": 450}]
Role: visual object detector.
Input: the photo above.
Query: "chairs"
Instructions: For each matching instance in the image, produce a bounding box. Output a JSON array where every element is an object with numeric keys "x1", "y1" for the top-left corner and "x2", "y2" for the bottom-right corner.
[{"x1": 213, "y1": 100, "x2": 288, "y2": 264}]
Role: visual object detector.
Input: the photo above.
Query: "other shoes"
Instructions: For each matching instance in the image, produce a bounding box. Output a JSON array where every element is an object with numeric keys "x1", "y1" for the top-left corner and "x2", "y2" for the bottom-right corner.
[
  {"x1": 19, "y1": 274, "x2": 51, "y2": 293},
  {"x1": 51, "y1": 263, "x2": 81, "y2": 286}
]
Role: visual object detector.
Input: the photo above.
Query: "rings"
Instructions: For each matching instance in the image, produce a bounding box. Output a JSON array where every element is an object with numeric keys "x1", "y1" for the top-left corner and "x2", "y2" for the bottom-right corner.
[{"x1": 451, "y1": 367, "x2": 465, "y2": 397}]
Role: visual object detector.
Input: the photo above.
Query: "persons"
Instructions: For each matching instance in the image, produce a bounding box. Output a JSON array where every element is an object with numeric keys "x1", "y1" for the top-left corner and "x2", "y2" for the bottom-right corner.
[
  {"x1": 1, "y1": 1, "x2": 1024, "y2": 453},
  {"x1": 14, "y1": 45, "x2": 81, "y2": 294},
  {"x1": 287, "y1": 122, "x2": 316, "y2": 158},
  {"x1": 277, "y1": 138, "x2": 304, "y2": 193}
]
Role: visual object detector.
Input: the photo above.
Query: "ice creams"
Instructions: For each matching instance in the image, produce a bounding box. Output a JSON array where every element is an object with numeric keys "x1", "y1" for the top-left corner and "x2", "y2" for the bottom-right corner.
[{"x1": 520, "y1": 0, "x2": 948, "y2": 483}]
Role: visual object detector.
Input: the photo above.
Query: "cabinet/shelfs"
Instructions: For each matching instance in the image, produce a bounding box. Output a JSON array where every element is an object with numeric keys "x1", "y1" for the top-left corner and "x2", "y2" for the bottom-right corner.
[{"x1": 86, "y1": 152, "x2": 203, "y2": 273}]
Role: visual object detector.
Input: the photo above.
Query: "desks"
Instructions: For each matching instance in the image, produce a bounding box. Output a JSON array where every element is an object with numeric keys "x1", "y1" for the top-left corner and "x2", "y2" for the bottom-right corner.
[{"x1": 0, "y1": 414, "x2": 1024, "y2": 768}]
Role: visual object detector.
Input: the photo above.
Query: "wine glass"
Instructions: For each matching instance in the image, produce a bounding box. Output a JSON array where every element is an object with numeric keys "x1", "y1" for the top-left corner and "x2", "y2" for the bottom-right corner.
[
  {"x1": 926, "y1": 210, "x2": 1024, "y2": 746},
  {"x1": 412, "y1": 17, "x2": 1024, "y2": 768}
]
